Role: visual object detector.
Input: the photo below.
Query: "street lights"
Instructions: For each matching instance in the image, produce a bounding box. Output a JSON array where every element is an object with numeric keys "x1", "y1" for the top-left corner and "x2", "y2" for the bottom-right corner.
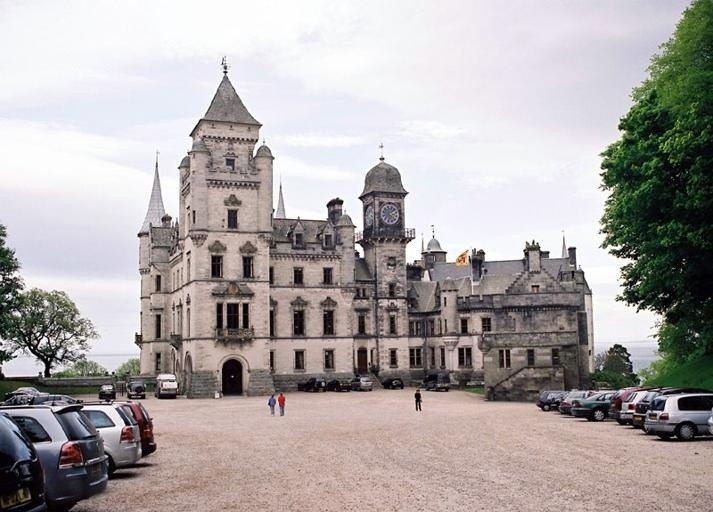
[{"x1": 373, "y1": 296, "x2": 412, "y2": 376}]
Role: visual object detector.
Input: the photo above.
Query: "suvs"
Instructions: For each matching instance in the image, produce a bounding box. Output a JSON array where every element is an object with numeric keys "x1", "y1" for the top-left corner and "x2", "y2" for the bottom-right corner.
[
  {"x1": 303, "y1": 378, "x2": 327, "y2": 392},
  {"x1": 127, "y1": 383, "x2": 145, "y2": 399},
  {"x1": 351, "y1": 373, "x2": 371, "y2": 390},
  {"x1": 382, "y1": 377, "x2": 404, "y2": 389}
]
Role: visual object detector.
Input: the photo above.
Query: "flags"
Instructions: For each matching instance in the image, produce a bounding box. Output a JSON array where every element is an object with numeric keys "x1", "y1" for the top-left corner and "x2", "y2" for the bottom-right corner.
[{"x1": 455, "y1": 250, "x2": 469, "y2": 267}]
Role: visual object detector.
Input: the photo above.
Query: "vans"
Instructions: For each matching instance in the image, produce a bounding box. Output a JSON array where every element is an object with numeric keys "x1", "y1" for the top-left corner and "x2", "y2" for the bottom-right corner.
[
  {"x1": 425, "y1": 371, "x2": 450, "y2": 391},
  {"x1": 536, "y1": 386, "x2": 712, "y2": 441}
]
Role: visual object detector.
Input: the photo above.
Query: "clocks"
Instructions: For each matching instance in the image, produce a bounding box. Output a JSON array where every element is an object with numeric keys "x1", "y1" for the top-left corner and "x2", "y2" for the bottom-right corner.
[
  {"x1": 381, "y1": 204, "x2": 400, "y2": 225},
  {"x1": 365, "y1": 205, "x2": 375, "y2": 227}
]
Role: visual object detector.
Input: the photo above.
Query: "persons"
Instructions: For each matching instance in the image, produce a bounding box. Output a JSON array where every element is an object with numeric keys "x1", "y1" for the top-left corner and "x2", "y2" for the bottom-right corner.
[
  {"x1": 268, "y1": 393, "x2": 276, "y2": 416},
  {"x1": 414, "y1": 388, "x2": 422, "y2": 411},
  {"x1": 278, "y1": 391, "x2": 285, "y2": 416}
]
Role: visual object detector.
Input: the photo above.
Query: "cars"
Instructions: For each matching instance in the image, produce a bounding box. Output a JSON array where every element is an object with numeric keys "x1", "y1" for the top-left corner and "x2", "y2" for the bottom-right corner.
[
  {"x1": 1, "y1": 387, "x2": 156, "y2": 512},
  {"x1": 100, "y1": 384, "x2": 115, "y2": 399},
  {"x1": 328, "y1": 379, "x2": 351, "y2": 392}
]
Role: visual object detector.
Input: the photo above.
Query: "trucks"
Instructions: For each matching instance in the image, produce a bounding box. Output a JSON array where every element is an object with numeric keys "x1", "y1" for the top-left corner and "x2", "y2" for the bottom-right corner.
[{"x1": 156, "y1": 374, "x2": 177, "y2": 398}]
